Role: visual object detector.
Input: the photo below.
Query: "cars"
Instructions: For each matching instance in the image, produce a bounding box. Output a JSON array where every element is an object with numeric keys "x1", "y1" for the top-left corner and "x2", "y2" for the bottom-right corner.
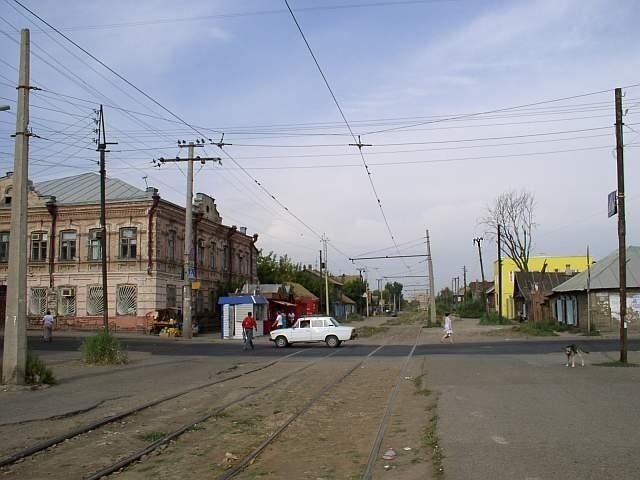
[{"x1": 268, "y1": 316, "x2": 357, "y2": 348}]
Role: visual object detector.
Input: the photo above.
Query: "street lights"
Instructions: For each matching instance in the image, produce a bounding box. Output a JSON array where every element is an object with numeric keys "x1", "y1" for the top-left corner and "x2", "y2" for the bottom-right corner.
[{"x1": 472, "y1": 237, "x2": 486, "y2": 312}]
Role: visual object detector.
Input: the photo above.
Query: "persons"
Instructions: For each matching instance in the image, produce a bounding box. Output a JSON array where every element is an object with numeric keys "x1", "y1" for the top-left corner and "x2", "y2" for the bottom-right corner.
[
  {"x1": 42, "y1": 310, "x2": 54, "y2": 341},
  {"x1": 302, "y1": 312, "x2": 305, "y2": 315},
  {"x1": 241, "y1": 312, "x2": 257, "y2": 350},
  {"x1": 272, "y1": 311, "x2": 283, "y2": 330},
  {"x1": 281, "y1": 310, "x2": 287, "y2": 328},
  {"x1": 440, "y1": 313, "x2": 454, "y2": 343},
  {"x1": 526, "y1": 312, "x2": 528, "y2": 319},
  {"x1": 288, "y1": 309, "x2": 295, "y2": 328},
  {"x1": 192, "y1": 315, "x2": 198, "y2": 326},
  {"x1": 520, "y1": 313, "x2": 524, "y2": 323}
]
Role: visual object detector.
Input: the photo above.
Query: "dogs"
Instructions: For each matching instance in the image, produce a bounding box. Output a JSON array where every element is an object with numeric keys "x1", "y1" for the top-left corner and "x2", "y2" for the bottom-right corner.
[{"x1": 560, "y1": 343, "x2": 590, "y2": 368}]
